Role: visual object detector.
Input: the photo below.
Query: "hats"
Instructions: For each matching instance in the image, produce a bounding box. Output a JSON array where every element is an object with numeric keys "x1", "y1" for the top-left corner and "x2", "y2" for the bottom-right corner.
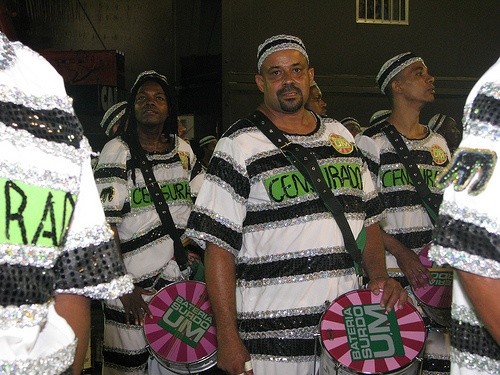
[
  {"x1": 129, "y1": 69, "x2": 170, "y2": 97},
  {"x1": 199, "y1": 136, "x2": 216, "y2": 147},
  {"x1": 99, "y1": 100, "x2": 128, "y2": 137},
  {"x1": 340, "y1": 117, "x2": 361, "y2": 129},
  {"x1": 427, "y1": 113, "x2": 447, "y2": 132},
  {"x1": 369, "y1": 109, "x2": 392, "y2": 127},
  {"x1": 257, "y1": 34, "x2": 310, "y2": 74},
  {"x1": 375, "y1": 51, "x2": 424, "y2": 96}
]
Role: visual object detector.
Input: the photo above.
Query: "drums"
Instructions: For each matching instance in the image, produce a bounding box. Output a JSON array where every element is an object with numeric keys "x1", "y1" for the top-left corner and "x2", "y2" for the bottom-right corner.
[
  {"x1": 143, "y1": 280, "x2": 226, "y2": 375},
  {"x1": 318, "y1": 289, "x2": 429, "y2": 375},
  {"x1": 413, "y1": 236, "x2": 454, "y2": 330}
]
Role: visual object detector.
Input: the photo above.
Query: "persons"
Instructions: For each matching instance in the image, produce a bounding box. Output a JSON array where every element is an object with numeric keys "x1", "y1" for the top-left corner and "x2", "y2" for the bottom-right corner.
[
  {"x1": 185, "y1": 33, "x2": 415, "y2": 375},
  {"x1": 0, "y1": 30, "x2": 219, "y2": 375},
  {"x1": 304, "y1": 52, "x2": 500, "y2": 375}
]
